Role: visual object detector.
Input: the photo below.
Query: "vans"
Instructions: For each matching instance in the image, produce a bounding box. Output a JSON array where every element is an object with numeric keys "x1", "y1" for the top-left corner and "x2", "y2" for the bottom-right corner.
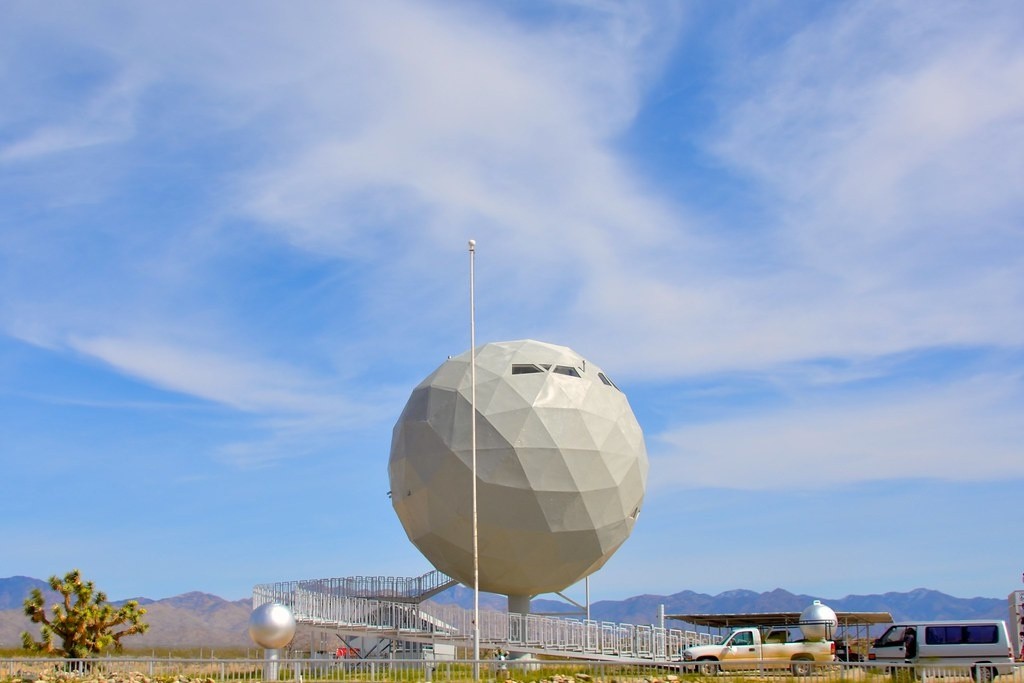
[{"x1": 860, "y1": 618, "x2": 1017, "y2": 683}]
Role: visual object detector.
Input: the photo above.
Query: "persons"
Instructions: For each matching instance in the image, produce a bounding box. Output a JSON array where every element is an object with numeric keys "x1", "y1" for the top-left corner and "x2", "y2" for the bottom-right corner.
[
  {"x1": 493, "y1": 647, "x2": 511, "y2": 680},
  {"x1": 731, "y1": 632, "x2": 753, "y2": 645},
  {"x1": 902, "y1": 627, "x2": 914, "y2": 652}
]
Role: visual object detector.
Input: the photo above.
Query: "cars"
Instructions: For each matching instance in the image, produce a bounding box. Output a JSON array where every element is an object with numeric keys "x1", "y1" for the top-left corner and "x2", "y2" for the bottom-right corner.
[
  {"x1": 849, "y1": 652, "x2": 864, "y2": 664},
  {"x1": 833, "y1": 639, "x2": 852, "y2": 662}
]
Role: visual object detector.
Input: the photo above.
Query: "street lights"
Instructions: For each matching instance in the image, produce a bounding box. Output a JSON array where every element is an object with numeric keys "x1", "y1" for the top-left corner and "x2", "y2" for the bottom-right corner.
[{"x1": 465, "y1": 237, "x2": 483, "y2": 683}]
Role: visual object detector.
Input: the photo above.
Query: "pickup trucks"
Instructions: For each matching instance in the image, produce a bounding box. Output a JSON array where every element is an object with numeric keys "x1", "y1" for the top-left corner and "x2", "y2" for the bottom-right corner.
[{"x1": 680, "y1": 626, "x2": 838, "y2": 678}]
